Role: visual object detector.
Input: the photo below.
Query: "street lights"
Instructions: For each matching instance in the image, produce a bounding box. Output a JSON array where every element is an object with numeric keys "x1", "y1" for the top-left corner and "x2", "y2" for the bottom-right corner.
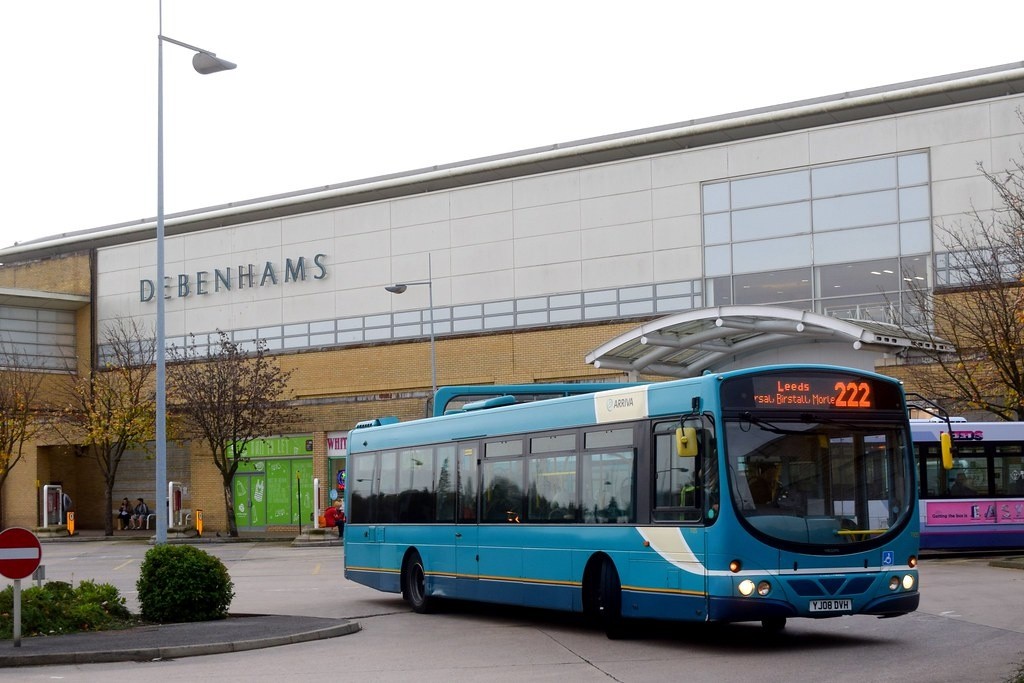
[{"x1": 154, "y1": 1, "x2": 238, "y2": 547}]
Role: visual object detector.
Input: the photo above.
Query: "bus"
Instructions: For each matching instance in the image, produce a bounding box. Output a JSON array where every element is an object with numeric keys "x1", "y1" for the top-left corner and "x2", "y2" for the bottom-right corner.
[
  {"x1": 809, "y1": 416, "x2": 1023, "y2": 553},
  {"x1": 341, "y1": 361, "x2": 919, "y2": 637}
]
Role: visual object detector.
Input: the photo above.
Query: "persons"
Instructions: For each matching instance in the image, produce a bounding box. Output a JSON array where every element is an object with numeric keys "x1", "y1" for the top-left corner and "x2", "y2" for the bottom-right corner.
[
  {"x1": 325, "y1": 499, "x2": 345, "y2": 539},
  {"x1": 118, "y1": 497, "x2": 149, "y2": 530},
  {"x1": 749, "y1": 468, "x2": 772, "y2": 504},
  {"x1": 951, "y1": 473, "x2": 976, "y2": 497}
]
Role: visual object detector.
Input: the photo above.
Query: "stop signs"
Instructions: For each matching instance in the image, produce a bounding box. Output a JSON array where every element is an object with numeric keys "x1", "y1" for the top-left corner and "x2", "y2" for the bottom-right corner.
[{"x1": 0, "y1": 527, "x2": 42, "y2": 580}]
